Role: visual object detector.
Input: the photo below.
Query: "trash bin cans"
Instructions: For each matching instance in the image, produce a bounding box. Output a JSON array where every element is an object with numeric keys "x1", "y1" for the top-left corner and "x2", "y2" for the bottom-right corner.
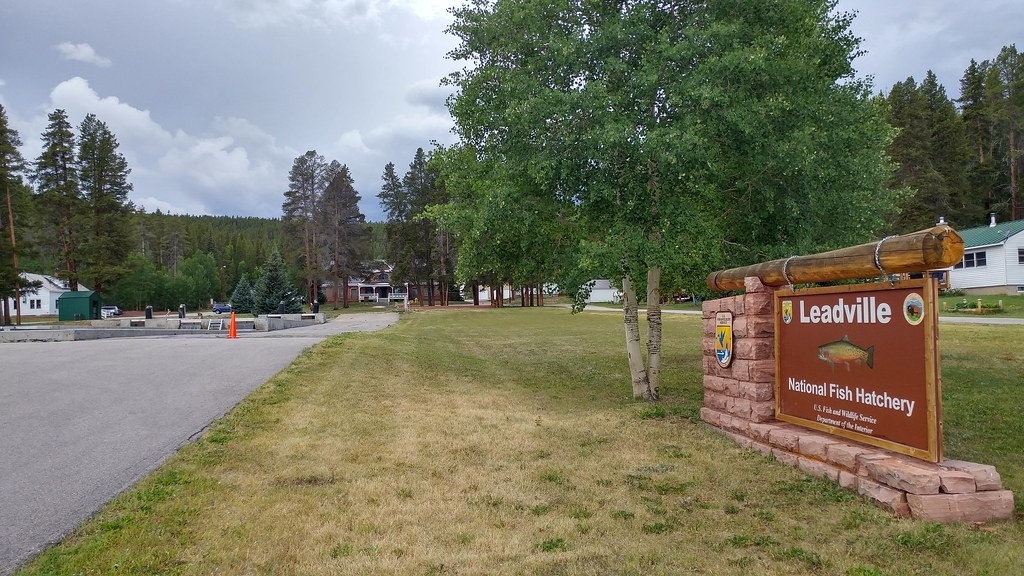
[
  {"x1": 179, "y1": 304, "x2": 186, "y2": 317},
  {"x1": 179, "y1": 306, "x2": 184, "y2": 318},
  {"x1": 146, "y1": 306, "x2": 153, "y2": 317},
  {"x1": 146, "y1": 308, "x2": 152, "y2": 319}
]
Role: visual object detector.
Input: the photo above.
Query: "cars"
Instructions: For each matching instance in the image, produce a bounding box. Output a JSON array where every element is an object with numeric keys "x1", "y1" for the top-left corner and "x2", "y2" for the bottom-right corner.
[
  {"x1": 101, "y1": 306, "x2": 120, "y2": 316},
  {"x1": 213, "y1": 303, "x2": 231, "y2": 315}
]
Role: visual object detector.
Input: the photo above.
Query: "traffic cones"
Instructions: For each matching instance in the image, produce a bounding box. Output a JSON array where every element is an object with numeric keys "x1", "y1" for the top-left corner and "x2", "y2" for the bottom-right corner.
[{"x1": 226, "y1": 312, "x2": 240, "y2": 339}]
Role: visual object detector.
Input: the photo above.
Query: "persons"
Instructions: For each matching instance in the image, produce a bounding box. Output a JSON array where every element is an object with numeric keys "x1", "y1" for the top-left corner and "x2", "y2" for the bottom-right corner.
[
  {"x1": 310, "y1": 298, "x2": 319, "y2": 313},
  {"x1": 277, "y1": 301, "x2": 285, "y2": 314}
]
